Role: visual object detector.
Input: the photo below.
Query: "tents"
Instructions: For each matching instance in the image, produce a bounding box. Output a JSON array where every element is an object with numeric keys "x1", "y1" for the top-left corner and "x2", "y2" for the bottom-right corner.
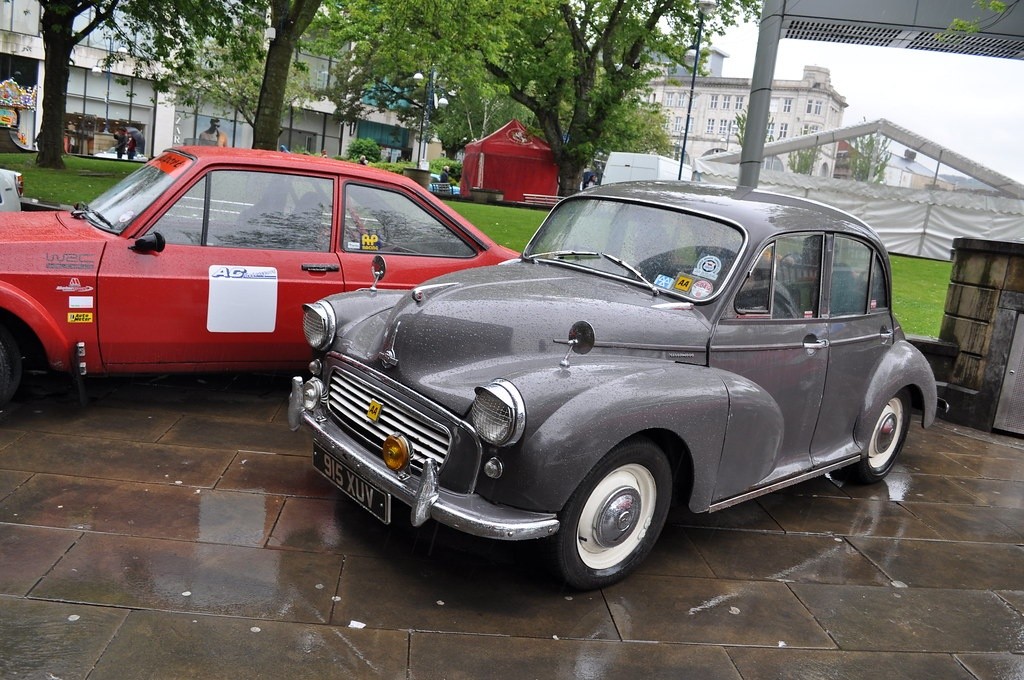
[{"x1": 460, "y1": 119, "x2": 561, "y2": 203}]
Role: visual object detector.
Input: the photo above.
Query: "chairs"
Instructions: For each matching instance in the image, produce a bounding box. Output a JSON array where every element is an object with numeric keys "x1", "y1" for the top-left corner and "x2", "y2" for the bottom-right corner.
[
  {"x1": 275, "y1": 192, "x2": 325, "y2": 252},
  {"x1": 236, "y1": 180, "x2": 287, "y2": 227}
]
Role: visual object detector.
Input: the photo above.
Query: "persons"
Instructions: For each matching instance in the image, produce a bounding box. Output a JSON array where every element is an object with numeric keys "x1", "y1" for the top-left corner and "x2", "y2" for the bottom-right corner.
[
  {"x1": 584, "y1": 175, "x2": 598, "y2": 189},
  {"x1": 321, "y1": 149, "x2": 327, "y2": 158},
  {"x1": 357, "y1": 154, "x2": 367, "y2": 165},
  {"x1": 127, "y1": 136, "x2": 137, "y2": 160},
  {"x1": 440, "y1": 166, "x2": 449, "y2": 183},
  {"x1": 198, "y1": 117, "x2": 228, "y2": 147},
  {"x1": 280, "y1": 144, "x2": 291, "y2": 153},
  {"x1": 114, "y1": 126, "x2": 127, "y2": 159}
]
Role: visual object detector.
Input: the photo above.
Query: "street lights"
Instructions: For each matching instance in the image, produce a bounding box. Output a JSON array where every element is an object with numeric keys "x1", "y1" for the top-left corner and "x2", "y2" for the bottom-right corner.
[
  {"x1": 677, "y1": 0, "x2": 718, "y2": 180},
  {"x1": 414, "y1": 64, "x2": 448, "y2": 173},
  {"x1": 90, "y1": 35, "x2": 126, "y2": 136}
]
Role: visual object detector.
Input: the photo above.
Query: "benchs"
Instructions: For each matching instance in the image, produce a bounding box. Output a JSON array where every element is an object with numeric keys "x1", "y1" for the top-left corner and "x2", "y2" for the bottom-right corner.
[
  {"x1": 431, "y1": 183, "x2": 460, "y2": 198},
  {"x1": 469, "y1": 188, "x2": 504, "y2": 201},
  {"x1": 523, "y1": 194, "x2": 567, "y2": 206}
]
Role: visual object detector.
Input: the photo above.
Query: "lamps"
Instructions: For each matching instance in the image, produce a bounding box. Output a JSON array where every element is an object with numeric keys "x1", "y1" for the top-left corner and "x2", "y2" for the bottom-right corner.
[{"x1": 346, "y1": 120, "x2": 357, "y2": 136}]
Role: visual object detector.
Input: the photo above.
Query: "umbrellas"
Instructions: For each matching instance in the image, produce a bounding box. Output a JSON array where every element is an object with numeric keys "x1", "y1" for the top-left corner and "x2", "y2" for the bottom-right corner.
[{"x1": 125, "y1": 126, "x2": 145, "y2": 154}]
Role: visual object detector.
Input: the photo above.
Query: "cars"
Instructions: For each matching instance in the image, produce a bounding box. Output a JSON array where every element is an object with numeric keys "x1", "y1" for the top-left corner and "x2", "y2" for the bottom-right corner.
[
  {"x1": 284, "y1": 181, "x2": 941, "y2": 593},
  {"x1": 428, "y1": 174, "x2": 461, "y2": 194},
  {"x1": 0, "y1": 146, "x2": 523, "y2": 407}
]
